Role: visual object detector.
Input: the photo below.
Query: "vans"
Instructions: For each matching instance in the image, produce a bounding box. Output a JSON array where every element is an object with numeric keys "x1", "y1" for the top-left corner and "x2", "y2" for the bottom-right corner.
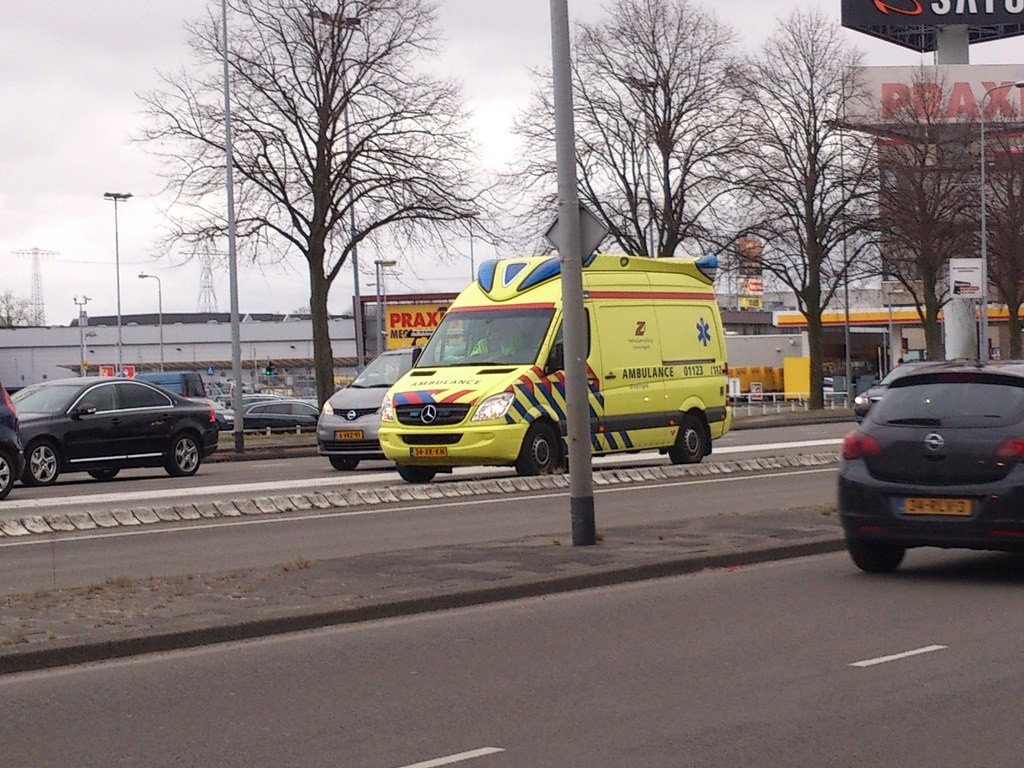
[{"x1": 131, "y1": 370, "x2": 209, "y2": 402}]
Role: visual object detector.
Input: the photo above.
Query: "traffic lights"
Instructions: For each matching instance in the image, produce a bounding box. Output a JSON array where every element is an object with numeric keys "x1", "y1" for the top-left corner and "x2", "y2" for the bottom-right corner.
[{"x1": 265, "y1": 367, "x2": 274, "y2": 376}]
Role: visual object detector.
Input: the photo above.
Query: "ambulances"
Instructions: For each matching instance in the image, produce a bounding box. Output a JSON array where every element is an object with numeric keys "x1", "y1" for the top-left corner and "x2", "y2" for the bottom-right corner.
[{"x1": 377, "y1": 253, "x2": 733, "y2": 488}]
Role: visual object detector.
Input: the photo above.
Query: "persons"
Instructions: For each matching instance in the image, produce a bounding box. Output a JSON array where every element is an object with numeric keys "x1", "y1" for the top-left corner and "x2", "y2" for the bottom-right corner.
[
  {"x1": 892, "y1": 358, "x2": 907, "y2": 370},
  {"x1": 471, "y1": 318, "x2": 524, "y2": 358}
]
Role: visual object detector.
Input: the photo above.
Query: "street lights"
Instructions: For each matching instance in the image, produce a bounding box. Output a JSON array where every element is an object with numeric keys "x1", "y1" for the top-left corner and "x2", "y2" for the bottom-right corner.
[
  {"x1": 137, "y1": 274, "x2": 163, "y2": 373},
  {"x1": 976, "y1": 79, "x2": 1024, "y2": 357},
  {"x1": 101, "y1": 192, "x2": 133, "y2": 376}
]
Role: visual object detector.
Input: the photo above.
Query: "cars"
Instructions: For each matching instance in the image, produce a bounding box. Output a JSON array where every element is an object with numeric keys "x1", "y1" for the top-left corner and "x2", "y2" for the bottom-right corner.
[
  {"x1": 0, "y1": 379, "x2": 27, "y2": 501},
  {"x1": 181, "y1": 397, "x2": 239, "y2": 433},
  {"x1": 836, "y1": 357, "x2": 1024, "y2": 574},
  {"x1": 225, "y1": 391, "x2": 284, "y2": 412},
  {"x1": 239, "y1": 399, "x2": 321, "y2": 432},
  {"x1": 849, "y1": 358, "x2": 945, "y2": 425},
  {"x1": 316, "y1": 343, "x2": 463, "y2": 474},
  {"x1": 9, "y1": 376, "x2": 222, "y2": 488}
]
